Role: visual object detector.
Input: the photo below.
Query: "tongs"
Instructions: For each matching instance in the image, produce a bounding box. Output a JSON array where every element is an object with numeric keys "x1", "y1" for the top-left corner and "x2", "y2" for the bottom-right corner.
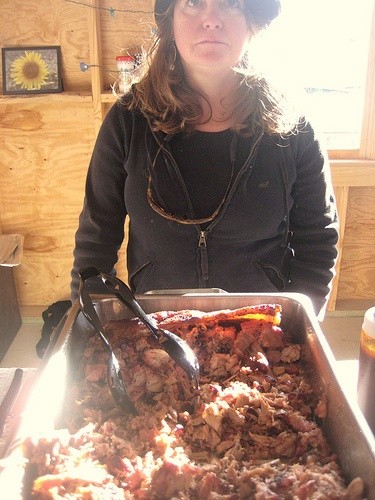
[{"x1": 80, "y1": 266, "x2": 201, "y2": 413}]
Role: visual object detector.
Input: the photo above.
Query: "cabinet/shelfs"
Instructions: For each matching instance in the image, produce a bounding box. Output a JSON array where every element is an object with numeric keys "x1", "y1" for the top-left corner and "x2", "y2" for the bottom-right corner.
[
  {"x1": 0, "y1": 1, "x2": 375, "y2": 324},
  {"x1": 0, "y1": 266, "x2": 22, "y2": 363}
]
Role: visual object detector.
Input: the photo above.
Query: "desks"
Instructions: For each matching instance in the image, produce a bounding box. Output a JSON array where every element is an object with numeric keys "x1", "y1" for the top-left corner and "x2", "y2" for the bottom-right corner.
[{"x1": 0, "y1": 359, "x2": 360, "y2": 463}]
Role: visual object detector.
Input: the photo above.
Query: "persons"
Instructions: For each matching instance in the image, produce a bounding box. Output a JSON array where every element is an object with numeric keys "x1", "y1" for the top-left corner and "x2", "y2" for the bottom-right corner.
[{"x1": 69, "y1": 0, "x2": 338, "y2": 316}]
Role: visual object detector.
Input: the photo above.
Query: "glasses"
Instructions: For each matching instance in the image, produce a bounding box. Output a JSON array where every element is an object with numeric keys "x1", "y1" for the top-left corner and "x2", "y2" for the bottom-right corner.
[{"x1": 147, "y1": 139, "x2": 235, "y2": 224}]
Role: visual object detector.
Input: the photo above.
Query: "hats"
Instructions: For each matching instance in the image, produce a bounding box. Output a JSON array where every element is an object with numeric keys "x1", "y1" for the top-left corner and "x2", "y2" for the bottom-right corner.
[{"x1": 153, "y1": 0, "x2": 279, "y2": 27}]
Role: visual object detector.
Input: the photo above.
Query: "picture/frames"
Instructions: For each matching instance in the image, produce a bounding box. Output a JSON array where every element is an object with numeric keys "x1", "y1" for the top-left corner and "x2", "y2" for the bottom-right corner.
[{"x1": 1, "y1": 45, "x2": 63, "y2": 95}]
